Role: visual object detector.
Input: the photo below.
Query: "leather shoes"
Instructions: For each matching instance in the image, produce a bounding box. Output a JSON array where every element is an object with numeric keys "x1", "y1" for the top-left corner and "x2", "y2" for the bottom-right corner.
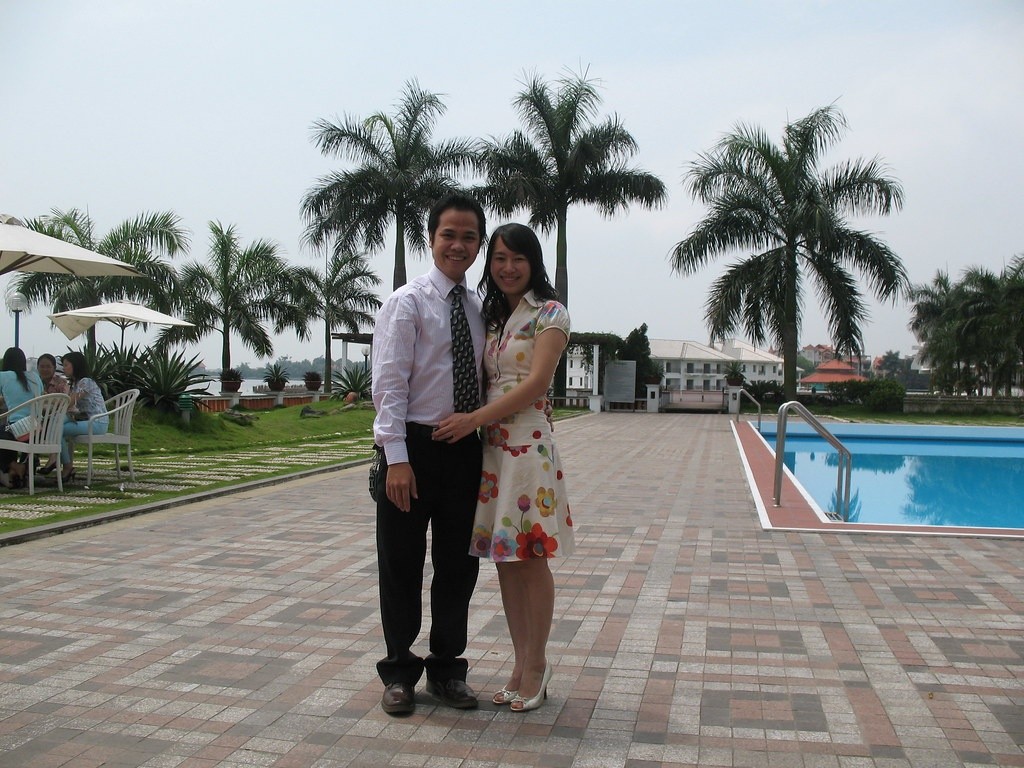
[
  {"x1": 426, "y1": 670, "x2": 478, "y2": 708},
  {"x1": 382, "y1": 681, "x2": 415, "y2": 712}
]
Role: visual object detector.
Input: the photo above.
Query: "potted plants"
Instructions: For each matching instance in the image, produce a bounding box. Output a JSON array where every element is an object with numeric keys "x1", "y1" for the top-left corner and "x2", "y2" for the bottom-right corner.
[
  {"x1": 217, "y1": 367, "x2": 245, "y2": 392},
  {"x1": 302, "y1": 370, "x2": 322, "y2": 392},
  {"x1": 262, "y1": 362, "x2": 292, "y2": 391},
  {"x1": 643, "y1": 359, "x2": 666, "y2": 384},
  {"x1": 721, "y1": 358, "x2": 748, "y2": 385}
]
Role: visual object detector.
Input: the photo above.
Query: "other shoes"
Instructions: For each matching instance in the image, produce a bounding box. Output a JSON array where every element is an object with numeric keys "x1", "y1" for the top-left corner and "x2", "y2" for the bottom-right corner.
[{"x1": 18, "y1": 464, "x2": 25, "y2": 486}]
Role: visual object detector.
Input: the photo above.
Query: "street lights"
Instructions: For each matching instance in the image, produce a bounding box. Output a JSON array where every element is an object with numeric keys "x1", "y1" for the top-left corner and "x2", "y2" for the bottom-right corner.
[
  {"x1": 361, "y1": 345, "x2": 370, "y2": 386},
  {"x1": 6, "y1": 292, "x2": 28, "y2": 348}
]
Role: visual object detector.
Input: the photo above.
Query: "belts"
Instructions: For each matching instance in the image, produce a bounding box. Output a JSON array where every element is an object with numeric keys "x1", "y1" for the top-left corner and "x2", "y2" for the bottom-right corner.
[{"x1": 406, "y1": 421, "x2": 440, "y2": 440}]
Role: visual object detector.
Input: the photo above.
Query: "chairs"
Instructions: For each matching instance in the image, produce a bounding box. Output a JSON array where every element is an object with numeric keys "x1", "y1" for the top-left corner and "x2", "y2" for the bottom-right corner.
[
  {"x1": 0, "y1": 392, "x2": 73, "y2": 496},
  {"x1": 69, "y1": 388, "x2": 141, "y2": 490}
]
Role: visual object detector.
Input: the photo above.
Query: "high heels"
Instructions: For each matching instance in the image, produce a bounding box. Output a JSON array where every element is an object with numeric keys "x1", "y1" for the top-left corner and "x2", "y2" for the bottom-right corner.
[
  {"x1": 493, "y1": 657, "x2": 552, "y2": 711},
  {"x1": 56, "y1": 467, "x2": 76, "y2": 483},
  {"x1": 36, "y1": 461, "x2": 61, "y2": 474}
]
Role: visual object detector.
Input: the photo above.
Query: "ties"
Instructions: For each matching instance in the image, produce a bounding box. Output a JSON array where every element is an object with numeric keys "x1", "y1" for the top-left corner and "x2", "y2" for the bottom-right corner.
[{"x1": 449, "y1": 285, "x2": 480, "y2": 414}]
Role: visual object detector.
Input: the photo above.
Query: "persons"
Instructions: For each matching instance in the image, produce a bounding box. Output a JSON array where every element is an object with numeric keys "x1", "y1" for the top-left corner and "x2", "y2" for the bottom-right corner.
[
  {"x1": 369, "y1": 190, "x2": 486, "y2": 713},
  {"x1": 431, "y1": 223, "x2": 577, "y2": 711},
  {"x1": 0, "y1": 347, "x2": 109, "y2": 489}
]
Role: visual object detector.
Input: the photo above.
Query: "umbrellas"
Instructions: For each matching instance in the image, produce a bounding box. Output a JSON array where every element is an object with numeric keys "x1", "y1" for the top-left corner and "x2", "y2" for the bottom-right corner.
[
  {"x1": 46, "y1": 298, "x2": 196, "y2": 352},
  {"x1": 0, "y1": 212, "x2": 148, "y2": 277}
]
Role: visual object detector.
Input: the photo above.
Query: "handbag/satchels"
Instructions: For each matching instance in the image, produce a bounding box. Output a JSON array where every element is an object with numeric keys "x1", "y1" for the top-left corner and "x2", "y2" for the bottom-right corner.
[{"x1": 6, "y1": 415, "x2": 40, "y2": 441}]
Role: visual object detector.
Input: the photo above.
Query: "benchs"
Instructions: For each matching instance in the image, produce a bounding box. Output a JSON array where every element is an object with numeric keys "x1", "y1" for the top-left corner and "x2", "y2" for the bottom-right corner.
[{"x1": 549, "y1": 396, "x2": 588, "y2": 407}]
